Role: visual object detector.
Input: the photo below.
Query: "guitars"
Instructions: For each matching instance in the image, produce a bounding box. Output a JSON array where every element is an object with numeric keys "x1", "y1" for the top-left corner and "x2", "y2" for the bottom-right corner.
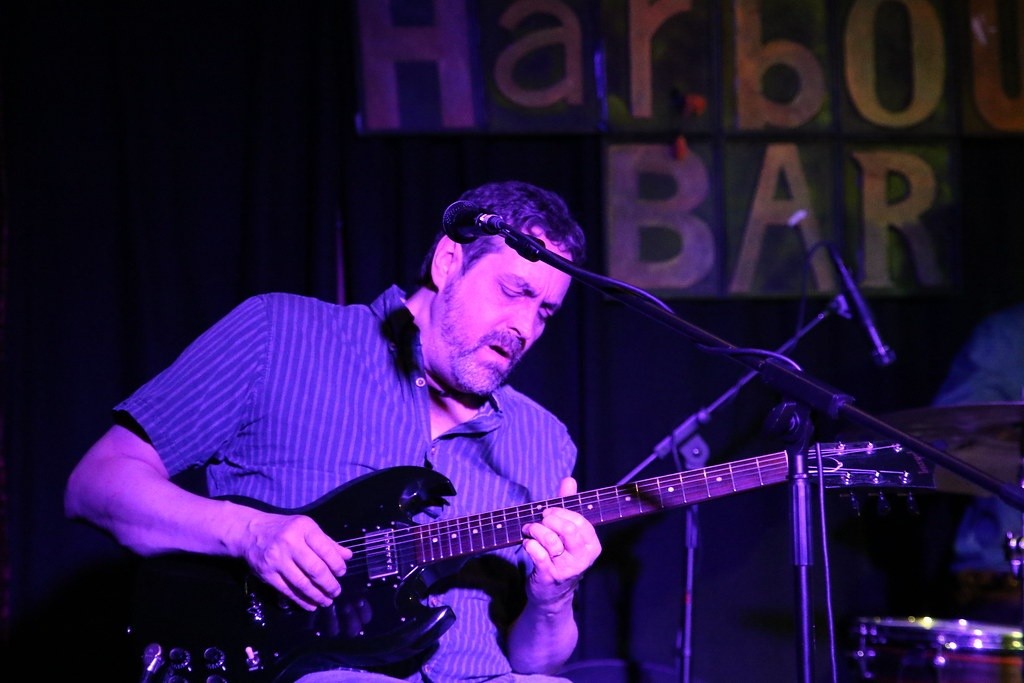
[{"x1": 84, "y1": 422, "x2": 951, "y2": 683}]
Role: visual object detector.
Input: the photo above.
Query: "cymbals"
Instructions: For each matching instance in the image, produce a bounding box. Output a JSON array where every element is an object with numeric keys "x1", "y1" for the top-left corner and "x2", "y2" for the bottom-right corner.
[{"x1": 857, "y1": 399, "x2": 1023, "y2": 497}]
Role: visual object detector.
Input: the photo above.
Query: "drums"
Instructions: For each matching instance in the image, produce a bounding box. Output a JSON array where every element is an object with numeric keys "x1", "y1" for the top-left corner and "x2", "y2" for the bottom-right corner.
[{"x1": 840, "y1": 607, "x2": 1024, "y2": 683}]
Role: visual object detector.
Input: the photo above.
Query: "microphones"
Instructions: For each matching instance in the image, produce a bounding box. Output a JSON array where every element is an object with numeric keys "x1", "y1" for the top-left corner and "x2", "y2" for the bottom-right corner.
[
  {"x1": 443, "y1": 199, "x2": 503, "y2": 245},
  {"x1": 826, "y1": 241, "x2": 895, "y2": 367}
]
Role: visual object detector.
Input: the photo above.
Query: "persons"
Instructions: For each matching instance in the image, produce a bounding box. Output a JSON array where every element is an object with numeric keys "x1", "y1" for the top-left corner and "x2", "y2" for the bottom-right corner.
[
  {"x1": 68, "y1": 185, "x2": 603, "y2": 683},
  {"x1": 935, "y1": 310, "x2": 1024, "y2": 634}
]
